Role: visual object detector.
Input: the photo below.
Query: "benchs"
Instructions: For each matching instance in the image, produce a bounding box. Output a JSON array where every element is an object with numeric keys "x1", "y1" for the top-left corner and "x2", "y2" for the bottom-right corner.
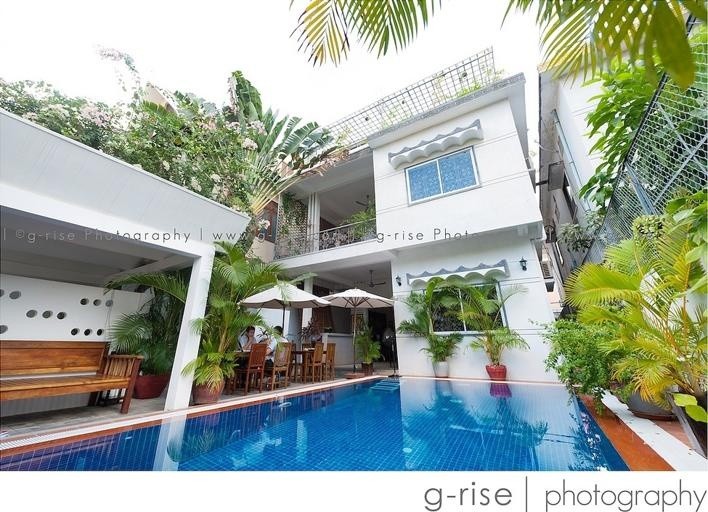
[{"x1": 0, "y1": 341, "x2": 142, "y2": 413}]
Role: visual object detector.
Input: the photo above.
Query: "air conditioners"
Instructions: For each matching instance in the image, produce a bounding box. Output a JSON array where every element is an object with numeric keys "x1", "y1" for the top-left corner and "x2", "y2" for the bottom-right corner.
[{"x1": 541, "y1": 249, "x2": 553, "y2": 279}]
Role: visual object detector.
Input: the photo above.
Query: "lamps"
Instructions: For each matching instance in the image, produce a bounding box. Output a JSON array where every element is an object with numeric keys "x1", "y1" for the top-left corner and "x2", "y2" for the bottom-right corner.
[
  {"x1": 395, "y1": 276, "x2": 403, "y2": 289},
  {"x1": 520, "y1": 257, "x2": 527, "y2": 271}
]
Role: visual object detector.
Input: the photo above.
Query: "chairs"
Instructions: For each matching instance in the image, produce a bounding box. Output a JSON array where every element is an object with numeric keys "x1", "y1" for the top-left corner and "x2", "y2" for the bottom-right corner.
[{"x1": 236, "y1": 338, "x2": 336, "y2": 396}]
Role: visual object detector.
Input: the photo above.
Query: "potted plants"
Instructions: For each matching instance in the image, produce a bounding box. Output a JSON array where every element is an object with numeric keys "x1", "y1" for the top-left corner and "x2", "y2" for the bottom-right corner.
[
  {"x1": 489, "y1": 383, "x2": 547, "y2": 471},
  {"x1": 391, "y1": 277, "x2": 461, "y2": 378},
  {"x1": 114, "y1": 288, "x2": 185, "y2": 397},
  {"x1": 166, "y1": 414, "x2": 236, "y2": 462},
  {"x1": 109, "y1": 238, "x2": 316, "y2": 403},
  {"x1": 540, "y1": 319, "x2": 678, "y2": 420},
  {"x1": 354, "y1": 326, "x2": 383, "y2": 375},
  {"x1": 446, "y1": 280, "x2": 530, "y2": 382},
  {"x1": 408, "y1": 381, "x2": 481, "y2": 471}
]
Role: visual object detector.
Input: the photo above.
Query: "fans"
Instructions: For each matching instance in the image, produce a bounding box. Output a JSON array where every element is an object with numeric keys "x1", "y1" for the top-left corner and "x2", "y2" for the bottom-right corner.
[
  {"x1": 357, "y1": 270, "x2": 386, "y2": 288},
  {"x1": 354, "y1": 195, "x2": 375, "y2": 214}
]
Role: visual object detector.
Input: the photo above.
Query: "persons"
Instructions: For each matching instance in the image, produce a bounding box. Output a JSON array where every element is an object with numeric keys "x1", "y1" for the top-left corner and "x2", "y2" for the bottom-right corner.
[
  {"x1": 257, "y1": 326, "x2": 288, "y2": 390},
  {"x1": 236, "y1": 325, "x2": 257, "y2": 389}
]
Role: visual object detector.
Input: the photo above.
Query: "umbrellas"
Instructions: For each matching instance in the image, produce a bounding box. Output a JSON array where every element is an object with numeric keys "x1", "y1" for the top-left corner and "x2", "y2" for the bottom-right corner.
[
  {"x1": 319, "y1": 287, "x2": 393, "y2": 374},
  {"x1": 237, "y1": 281, "x2": 332, "y2": 338}
]
